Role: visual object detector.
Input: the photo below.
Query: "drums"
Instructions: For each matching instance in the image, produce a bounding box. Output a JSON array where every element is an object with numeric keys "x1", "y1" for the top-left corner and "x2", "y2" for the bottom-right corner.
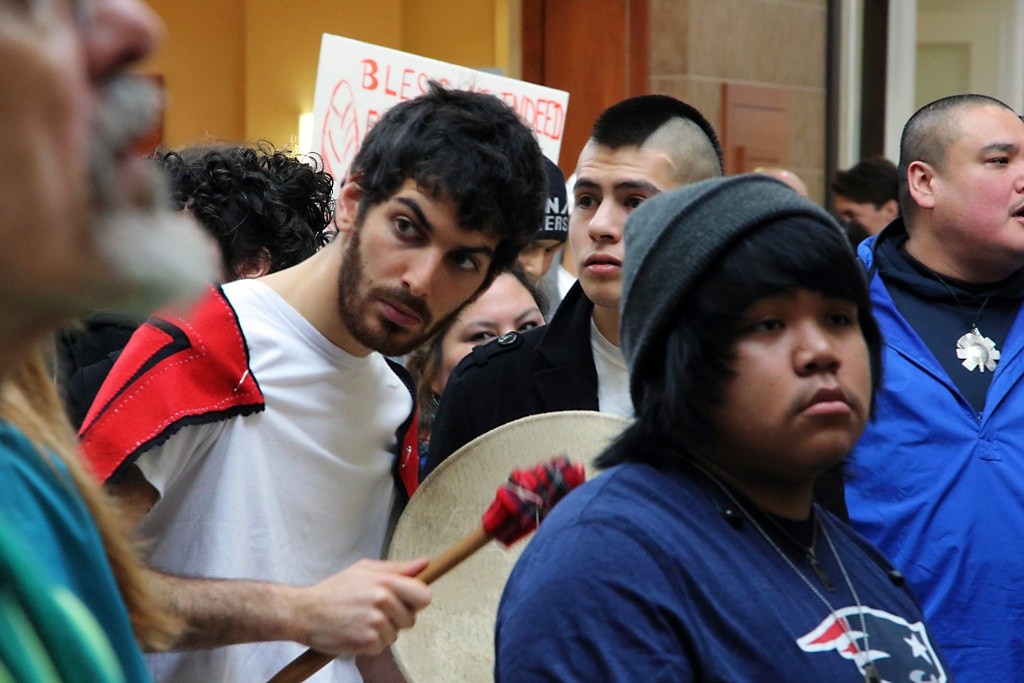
[{"x1": 267, "y1": 409, "x2": 633, "y2": 683}]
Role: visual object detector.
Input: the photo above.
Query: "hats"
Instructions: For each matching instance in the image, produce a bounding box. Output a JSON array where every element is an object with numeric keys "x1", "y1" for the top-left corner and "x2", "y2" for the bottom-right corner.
[
  {"x1": 617, "y1": 171, "x2": 852, "y2": 412},
  {"x1": 532, "y1": 155, "x2": 570, "y2": 243}
]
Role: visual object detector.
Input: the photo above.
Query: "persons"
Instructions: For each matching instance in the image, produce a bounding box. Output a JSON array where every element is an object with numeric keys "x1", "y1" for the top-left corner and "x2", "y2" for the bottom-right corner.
[
  {"x1": 406, "y1": 264, "x2": 550, "y2": 466},
  {"x1": 66, "y1": 145, "x2": 332, "y2": 432},
  {"x1": 832, "y1": 158, "x2": 899, "y2": 235},
  {"x1": 516, "y1": 154, "x2": 569, "y2": 284},
  {"x1": 0, "y1": 0, "x2": 183, "y2": 683},
  {"x1": 69, "y1": 85, "x2": 547, "y2": 683},
  {"x1": 817, "y1": 93, "x2": 1023, "y2": 682},
  {"x1": 755, "y1": 168, "x2": 806, "y2": 196},
  {"x1": 494, "y1": 175, "x2": 955, "y2": 683},
  {"x1": 424, "y1": 94, "x2": 723, "y2": 481}
]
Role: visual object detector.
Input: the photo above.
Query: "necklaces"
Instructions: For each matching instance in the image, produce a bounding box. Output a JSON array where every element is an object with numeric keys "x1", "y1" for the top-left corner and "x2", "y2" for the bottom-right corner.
[
  {"x1": 930, "y1": 268, "x2": 1001, "y2": 372},
  {"x1": 666, "y1": 449, "x2": 883, "y2": 683}
]
